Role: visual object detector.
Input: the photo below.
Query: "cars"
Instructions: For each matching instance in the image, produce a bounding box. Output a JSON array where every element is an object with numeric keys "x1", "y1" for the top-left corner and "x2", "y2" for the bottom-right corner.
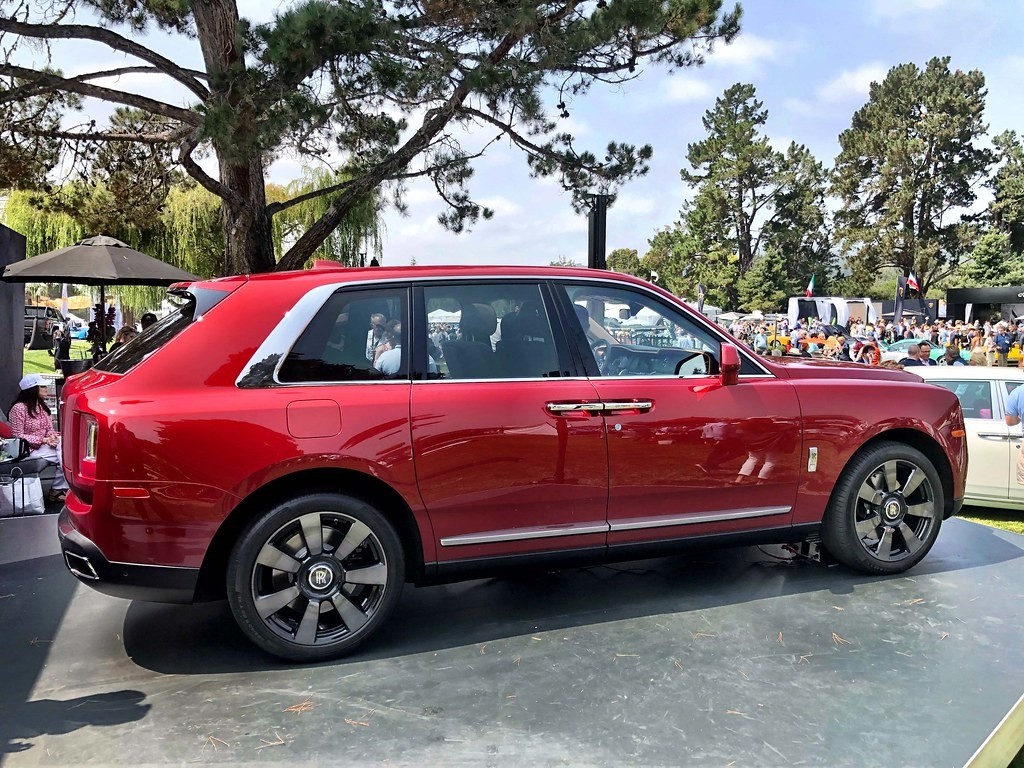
[
  {"x1": 69, "y1": 327, "x2": 88, "y2": 340},
  {"x1": 900, "y1": 363, "x2": 1024, "y2": 512},
  {"x1": 877, "y1": 338, "x2": 1024, "y2": 367}
]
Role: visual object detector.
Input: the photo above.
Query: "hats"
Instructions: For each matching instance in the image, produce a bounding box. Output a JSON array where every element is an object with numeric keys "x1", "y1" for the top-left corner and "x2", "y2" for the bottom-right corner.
[
  {"x1": 142, "y1": 313, "x2": 156, "y2": 322},
  {"x1": 19, "y1": 374, "x2": 52, "y2": 390}
]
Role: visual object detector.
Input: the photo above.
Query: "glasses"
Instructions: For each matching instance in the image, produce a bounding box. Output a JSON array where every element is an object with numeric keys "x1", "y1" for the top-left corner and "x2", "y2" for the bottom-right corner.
[{"x1": 39, "y1": 386, "x2": 46, "y2": 388}]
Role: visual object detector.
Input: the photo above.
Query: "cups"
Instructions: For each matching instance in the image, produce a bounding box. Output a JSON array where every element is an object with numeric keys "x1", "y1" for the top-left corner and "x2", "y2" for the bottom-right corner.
[{"x1": 3, "y1": 439, "x2": 19, "y2": 458}]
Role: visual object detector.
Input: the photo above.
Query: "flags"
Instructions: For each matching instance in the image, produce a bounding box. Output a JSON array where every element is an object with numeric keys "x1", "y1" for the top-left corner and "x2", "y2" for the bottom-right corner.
[
  {"x1": 806, "y1": 274, "x2": 814, "y2": 297},
  {"x1": 906, "y1": 272, "x2": 919, "y2": 292},
  {"x1": 651, "y1": 270, "x2": 659, "y2": 282}
]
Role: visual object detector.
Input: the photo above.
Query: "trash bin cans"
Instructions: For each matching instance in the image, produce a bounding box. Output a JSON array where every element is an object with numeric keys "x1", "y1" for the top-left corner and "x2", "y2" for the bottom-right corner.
[{"x1": 59, "y1": 359, "x2": 93, "y2": 382}]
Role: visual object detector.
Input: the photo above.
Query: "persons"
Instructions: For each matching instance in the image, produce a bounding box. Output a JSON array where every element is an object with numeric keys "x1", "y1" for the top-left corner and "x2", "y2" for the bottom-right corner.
[
  {"x1": 8, "y1": 373, "x2": 71, "y2": 503},
  {"x1": 572, "y1": 300, "x2": 1024, "y2": 375},
  {"x1": 367, "y1": 312, "x2": 461, "y2": 375},
  {"x1": 48, "y1": 312, "x2": 158, "y2": 379},
  {"x1": 1004, "y1": 384, "x2": 1024, "y2": 485}
]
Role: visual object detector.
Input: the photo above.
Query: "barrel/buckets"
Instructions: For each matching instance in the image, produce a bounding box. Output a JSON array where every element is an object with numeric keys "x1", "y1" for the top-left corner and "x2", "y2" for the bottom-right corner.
[{"x1": 60, "y1": 359, "x2": 93, "y2": 380}]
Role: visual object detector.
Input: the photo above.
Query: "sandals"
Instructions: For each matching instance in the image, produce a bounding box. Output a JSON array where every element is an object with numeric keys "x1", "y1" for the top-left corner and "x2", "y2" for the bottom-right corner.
[{"x1": 50, "y1": 491, "x2": 67, "y2": 502}]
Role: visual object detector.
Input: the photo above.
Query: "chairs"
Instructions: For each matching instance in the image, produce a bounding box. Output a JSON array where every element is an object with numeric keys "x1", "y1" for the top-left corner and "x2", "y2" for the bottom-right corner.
[
  {"x1": 503, "y1": 301, "x2": 554, "y2": 377},
  {"x1": 0, "y1": 408, "x2": 51, "y2": 514},
  {"x1": 442, "y1": 303, "x2": 498, "y2": 378}
]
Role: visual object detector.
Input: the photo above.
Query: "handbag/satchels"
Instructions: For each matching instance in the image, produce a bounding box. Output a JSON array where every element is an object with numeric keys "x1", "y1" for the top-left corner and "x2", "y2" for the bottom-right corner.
[
  {"x1": 0, "y1": 467, "x2": 45, "y2": 517},
  {"x1": 4, "y1": 438, "x2": 30, "y2": 463}
]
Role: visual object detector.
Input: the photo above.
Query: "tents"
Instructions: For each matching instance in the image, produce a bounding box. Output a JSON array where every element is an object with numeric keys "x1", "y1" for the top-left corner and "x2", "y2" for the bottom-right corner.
[{"x1": 427, "y1": 308, "x2": 462, "y2": 324}]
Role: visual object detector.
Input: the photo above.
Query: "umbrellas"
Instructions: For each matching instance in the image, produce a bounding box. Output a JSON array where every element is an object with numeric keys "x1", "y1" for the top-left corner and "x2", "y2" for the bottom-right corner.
[{"x1": 0, "y1": 232, "x2": 199, "y2": 354}]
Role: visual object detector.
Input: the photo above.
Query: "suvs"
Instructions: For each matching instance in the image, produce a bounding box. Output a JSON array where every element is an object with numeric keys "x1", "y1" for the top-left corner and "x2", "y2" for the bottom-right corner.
[{"x1": 56, "y1": 258, "x2": 969, "y2": 665}]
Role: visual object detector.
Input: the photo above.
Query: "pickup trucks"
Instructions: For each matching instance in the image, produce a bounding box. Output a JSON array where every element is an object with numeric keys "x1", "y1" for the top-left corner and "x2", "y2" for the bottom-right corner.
[{"x1": 24, "y1": 304, "x2": 71, "y2": 349}]
